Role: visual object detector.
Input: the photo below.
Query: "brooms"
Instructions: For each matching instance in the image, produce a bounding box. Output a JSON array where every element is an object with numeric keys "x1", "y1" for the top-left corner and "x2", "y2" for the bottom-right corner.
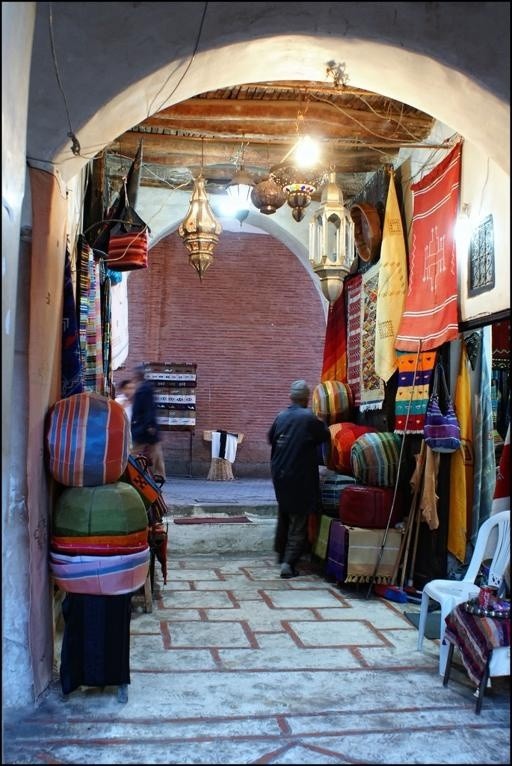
[{"x1": 373, "y1": 462, "x2": 423, "y2": 602}]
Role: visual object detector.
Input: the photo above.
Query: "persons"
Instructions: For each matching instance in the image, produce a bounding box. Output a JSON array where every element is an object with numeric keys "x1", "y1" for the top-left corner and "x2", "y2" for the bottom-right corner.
[
  {"x1": 265, "y1": 379, "x2": 331, "y2": 580},
  {"x1": 120, "y1": 363, "x2": 167, "y2": 482},
  {"x1": 113, "y1": 379, "x2": 137, "y2": 450}
]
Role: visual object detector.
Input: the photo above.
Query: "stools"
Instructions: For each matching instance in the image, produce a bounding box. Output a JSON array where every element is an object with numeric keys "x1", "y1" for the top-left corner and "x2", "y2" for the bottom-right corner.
[{"x1": 442, "y1": 600, "x2": 511, "y2": 717}]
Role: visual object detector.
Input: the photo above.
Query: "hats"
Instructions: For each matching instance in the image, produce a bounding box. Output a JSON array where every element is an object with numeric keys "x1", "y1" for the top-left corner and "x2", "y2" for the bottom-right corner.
[{"x1": 289, "y1": 379, "x2": 309, "y2": 397}]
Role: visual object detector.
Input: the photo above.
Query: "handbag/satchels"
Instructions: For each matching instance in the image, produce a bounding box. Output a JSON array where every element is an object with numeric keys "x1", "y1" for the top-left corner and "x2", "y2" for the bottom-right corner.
[{"x1": 423, "y1": 363, "x2": 461, "y2": 455}]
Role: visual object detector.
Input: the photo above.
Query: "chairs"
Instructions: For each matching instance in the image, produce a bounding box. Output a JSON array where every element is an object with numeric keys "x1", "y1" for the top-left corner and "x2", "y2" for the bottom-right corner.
[{"x1": 414, "y1": 510, "x2": 511, "y2": 677}]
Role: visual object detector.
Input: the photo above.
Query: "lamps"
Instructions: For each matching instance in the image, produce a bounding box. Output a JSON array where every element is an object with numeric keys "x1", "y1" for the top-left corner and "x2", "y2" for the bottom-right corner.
[{"x1": 177, "y1": 136, "x2": 361, "y2": 315}]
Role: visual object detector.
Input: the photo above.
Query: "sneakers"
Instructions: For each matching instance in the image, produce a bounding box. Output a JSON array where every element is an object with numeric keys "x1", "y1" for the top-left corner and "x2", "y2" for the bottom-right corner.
[{"x1": 280, "y1": 563, "x2": 299, "y2": 579}]
